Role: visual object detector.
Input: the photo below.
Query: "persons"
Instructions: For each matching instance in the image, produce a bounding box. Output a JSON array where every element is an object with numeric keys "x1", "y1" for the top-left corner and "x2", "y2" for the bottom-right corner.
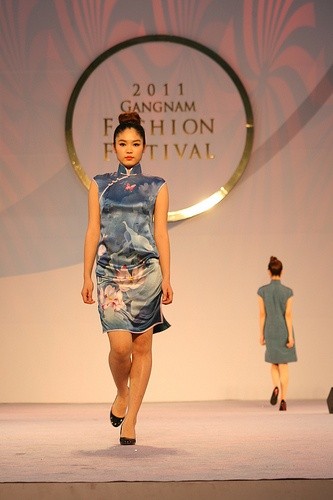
[
  {"x1": 256, "y1": 256, "x2": 298, "y2": 410},
  {"x1": 80, "y1": 112, "x2": 174, "y2": 445}
]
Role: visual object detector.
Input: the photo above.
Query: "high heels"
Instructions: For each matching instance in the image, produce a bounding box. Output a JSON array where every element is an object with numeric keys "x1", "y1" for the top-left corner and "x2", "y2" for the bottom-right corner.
[
  {"x1": 109, "y1": 394, "x2": 125, "y2": 427},
  {"x1": 119, "y1": 421, "x2": 136, "y2": 445},
  {"x1": 270, "y1": 386, "x2": 279, "y2": 405},
  {"x1": 279, "y1": 399, "x2": 287, "y2": 411}
]
